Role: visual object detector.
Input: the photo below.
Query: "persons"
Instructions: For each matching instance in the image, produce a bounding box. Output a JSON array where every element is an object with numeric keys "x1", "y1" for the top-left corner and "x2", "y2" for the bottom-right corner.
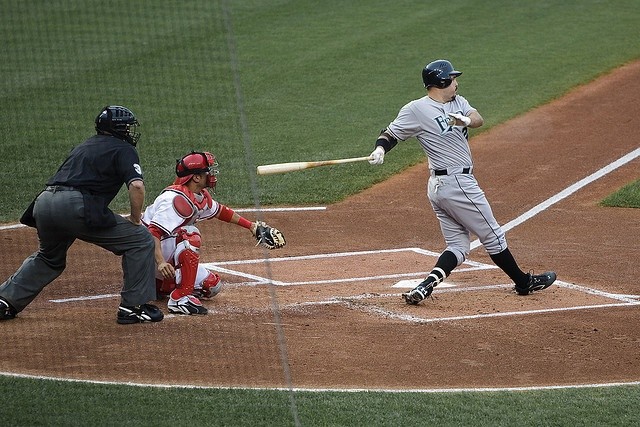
[
  {"x1": 368, "y1": 59, "x2": 556, "y2": 304},
  {"x1": 0, "y1": 104, "x2": 164, "y2": 324},
  {"x1": 141, "y1": 150, "x2": 286, "y2": 314}
]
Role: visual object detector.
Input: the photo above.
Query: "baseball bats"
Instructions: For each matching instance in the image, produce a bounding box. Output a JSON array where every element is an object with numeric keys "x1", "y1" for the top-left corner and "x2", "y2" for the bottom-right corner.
[{"x1": 257, "y1": 156, "x2": 375, "y2": 178}]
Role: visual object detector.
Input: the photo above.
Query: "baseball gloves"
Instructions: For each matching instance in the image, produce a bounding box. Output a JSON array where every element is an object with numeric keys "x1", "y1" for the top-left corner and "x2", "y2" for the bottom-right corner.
[{"x1": 254, "y1": 221, "x2": 287, "y2": 249}]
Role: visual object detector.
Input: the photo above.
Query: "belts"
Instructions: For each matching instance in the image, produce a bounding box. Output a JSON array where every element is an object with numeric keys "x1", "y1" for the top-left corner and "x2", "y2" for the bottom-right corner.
[
  {"x1": 46, "y1": 186, "x2": 81, "y2": 192},
  {"x1": 434, "y1": 168, "x2": 473, "y2": 175}
]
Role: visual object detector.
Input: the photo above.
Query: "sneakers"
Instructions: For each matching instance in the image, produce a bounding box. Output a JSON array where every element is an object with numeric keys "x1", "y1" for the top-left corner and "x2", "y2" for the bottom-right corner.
[
  {"x1": 515, "y1": 269, "x2": 556, "y2": 295},
  {"x1": 117, "y1": 303, "x2": 164, "y2": 323},
  {"x1": 401, "y1": 283, "x2": 433, "y2": 305},
  {"x1": 167, "y1": 295, "x2": 208, "y2": 314},
  {"x1": 0, "y1": 299, "x2": 14, "y2": 319}
]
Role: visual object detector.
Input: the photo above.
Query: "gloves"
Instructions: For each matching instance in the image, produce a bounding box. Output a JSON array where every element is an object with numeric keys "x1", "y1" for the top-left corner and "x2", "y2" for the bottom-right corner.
[
  {"x1": 448, "y1": 113, "x2": 471, "y2": 127},
  {"x1": 368, "y1": 146, "x2": 386, "y2": 165}
]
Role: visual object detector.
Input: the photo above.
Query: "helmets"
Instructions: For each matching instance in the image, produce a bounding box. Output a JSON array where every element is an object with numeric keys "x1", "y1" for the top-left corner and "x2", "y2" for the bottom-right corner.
[
  {"x1": 174, "y1": 152, "x2": 219, "y2": 188},
  {"x1": 422, "y1": 59, "x2": 463, "y2": 89},
  {"x1": 94, "y1": 105, "x2": 141, "y2": 146}
]
